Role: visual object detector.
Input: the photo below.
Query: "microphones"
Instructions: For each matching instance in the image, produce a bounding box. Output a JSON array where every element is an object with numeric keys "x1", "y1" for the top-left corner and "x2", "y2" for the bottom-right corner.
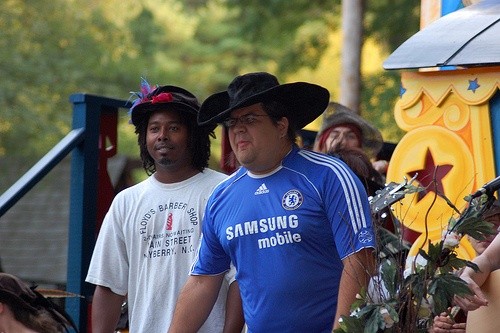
[{"x1": 471, "y1": 176, "x2": 500, "y2": 199}]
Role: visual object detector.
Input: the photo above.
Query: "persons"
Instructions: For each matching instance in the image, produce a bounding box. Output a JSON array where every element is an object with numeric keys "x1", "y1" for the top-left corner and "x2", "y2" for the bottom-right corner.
[
  {"x1": 84, "y1": 86, "x2": 246, "y2": 333},
  {"x1": 167, "y1": 72, "x2": 378, "y2": 333},
  {"x1": 297, "y1": 101, "x2": 422, "y2": 318},
  {"x1": 416, "y1": 199, "x2": 500, "y2": 333},
  {"x1": 0, "y1": 273, "x2": 82, "y2": 333}
]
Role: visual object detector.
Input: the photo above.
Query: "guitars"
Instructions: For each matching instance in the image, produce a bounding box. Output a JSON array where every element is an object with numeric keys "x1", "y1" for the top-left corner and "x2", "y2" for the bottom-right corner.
[{"x1": 367, "y1": 172, "x2": 419, "y2": 213}]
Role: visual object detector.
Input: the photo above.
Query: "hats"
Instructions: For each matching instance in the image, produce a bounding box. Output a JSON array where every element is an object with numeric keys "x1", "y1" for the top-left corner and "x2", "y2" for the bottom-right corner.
[
  {"x1": 312, "y1": 101, "x2": 384, "y2": 158},
  {"x1": 197, "y1": 73, "x2": 330, "y2": 138},
  {"x1": 129, "y1": 78, "x2": 200, "y2": 125}
]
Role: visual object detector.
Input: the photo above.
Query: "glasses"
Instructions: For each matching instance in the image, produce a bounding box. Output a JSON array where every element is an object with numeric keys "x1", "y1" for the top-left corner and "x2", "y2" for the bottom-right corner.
[{"x1": 223, "y1": 113, "x2": 271, "y2": 128}]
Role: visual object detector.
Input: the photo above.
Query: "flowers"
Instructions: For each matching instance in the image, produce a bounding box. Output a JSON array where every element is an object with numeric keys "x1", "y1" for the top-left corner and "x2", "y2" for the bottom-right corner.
[
  {"x1": 334, "y1": 194, "x2": 496, "y2": 333},
  {"x1": 124, "y1": 76, "x2": 174, "y2": 116}
]
